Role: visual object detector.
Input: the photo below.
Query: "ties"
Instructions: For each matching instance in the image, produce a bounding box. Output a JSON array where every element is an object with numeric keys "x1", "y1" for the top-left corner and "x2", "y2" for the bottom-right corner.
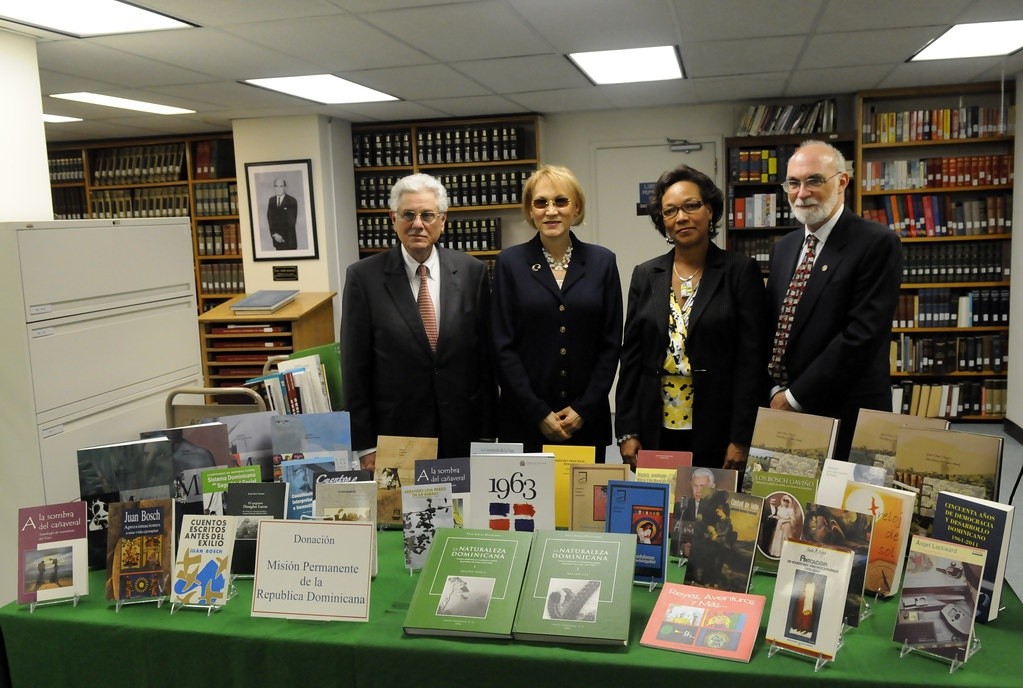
[
  {"x1": 278, "y1": 198, "x2": 280, "y2": 205},
  {"x1": 770, "y1": 235, "x2": 819, "y2": 386},
  {"x1": 417, "y1": 265, "x2": 438, "y2": 352}
]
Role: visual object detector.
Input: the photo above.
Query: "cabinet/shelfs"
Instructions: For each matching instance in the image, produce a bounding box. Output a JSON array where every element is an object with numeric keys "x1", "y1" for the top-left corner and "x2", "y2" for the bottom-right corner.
[
  {"x1": 0, "y1": 218, "x2": 201, "y2": 610},
  {"x1": 44, "y1": 133, "x2": 245, "y2": 312},
  {"x1": 721, "y1": 80, "x2": 1014, "y2": 425},
  {"x1": 198, "y1": 293, "x2": 337, "y2": 396},
  {"x1": 347, "y1": 113, "x2": 546, "y2": 289}
]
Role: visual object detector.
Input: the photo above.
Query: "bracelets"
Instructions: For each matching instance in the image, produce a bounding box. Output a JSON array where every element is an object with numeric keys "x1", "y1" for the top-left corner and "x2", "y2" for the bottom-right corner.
[{"x1": 617, "y1": 434, "x2": 638, "y2": 443}]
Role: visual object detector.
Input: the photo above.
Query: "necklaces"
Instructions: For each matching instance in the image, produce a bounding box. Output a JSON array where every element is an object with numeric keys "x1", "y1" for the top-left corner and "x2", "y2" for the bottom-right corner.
[
  {"x1": 674, "y1": 257, "x2": 703, "y2": 281},
  {"x1": 541, "y1": 241, "x2": 574, "y2": 270}
]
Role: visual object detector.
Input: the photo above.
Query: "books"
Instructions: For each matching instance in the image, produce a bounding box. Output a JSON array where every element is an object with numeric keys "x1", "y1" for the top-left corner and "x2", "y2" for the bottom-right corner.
[
  {"x1": 48, "y1": 141, "x2": 245, "y2": 315},
  {"x1": 728, "y1": 104, "x2": 1015, "y2": 418},
  {"x1": 211, "y1": 289, "x2": 299, "y2": 404},
  {"x1": 18, "y1": 355, "x2": 1014, "y2": 664},
  {"x1": 353, "y1": 127, "x2": 534, "y2": 251}
]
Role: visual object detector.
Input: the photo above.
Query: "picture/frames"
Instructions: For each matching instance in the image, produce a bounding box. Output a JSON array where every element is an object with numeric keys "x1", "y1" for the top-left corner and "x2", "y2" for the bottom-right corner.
[{"x1": 243, "y1": 160, "x2": 318, "y2": 261}]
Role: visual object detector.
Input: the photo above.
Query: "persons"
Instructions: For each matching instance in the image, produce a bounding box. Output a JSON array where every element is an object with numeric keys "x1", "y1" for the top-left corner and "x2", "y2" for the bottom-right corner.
[
  {"x1": 670, "y1": 469, "x2": 716, "y2": 558},
  {"x1": 491, "y1": 164, "x2": 623, "y2": 463},
  {"x1": 770, "y1": 495, "x2": 795, "y2": 558},
  {"x1": 615, "y1": 164, "x2": 777, "y2": 493},
  {"x1": 267, "y1": 177, "x2": 298, "y2": 250},
  {"x1": 340, "y1": 174, "x2": 499, "y2": 470},
  {"x1": 765, "y1": 140, "x2": 904, "y2": 462},
  {"x1": 762, "y1": 495, "x2": 777, "y2": 554}
]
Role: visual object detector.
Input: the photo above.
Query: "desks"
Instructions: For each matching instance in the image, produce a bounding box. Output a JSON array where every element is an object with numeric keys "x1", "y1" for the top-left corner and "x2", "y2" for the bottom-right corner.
[{"x1": 0, "y1": 525, "x2": 1023, "y2": 688}]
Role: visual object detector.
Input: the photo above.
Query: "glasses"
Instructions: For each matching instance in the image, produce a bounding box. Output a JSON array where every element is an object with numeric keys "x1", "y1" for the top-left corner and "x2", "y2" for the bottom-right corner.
[
  {"x1": 531, "y1": 197, "x2": 572, "y2": 209},
  {"x1": 780, "y1": 172, "x2": 842, "y2": 193},
  {"x1": 659, "y1": 199, "x2": 704, "y2": 219},
  {"x1": 395, "y1": 210, "x2": 441, "y2": 222}
]
